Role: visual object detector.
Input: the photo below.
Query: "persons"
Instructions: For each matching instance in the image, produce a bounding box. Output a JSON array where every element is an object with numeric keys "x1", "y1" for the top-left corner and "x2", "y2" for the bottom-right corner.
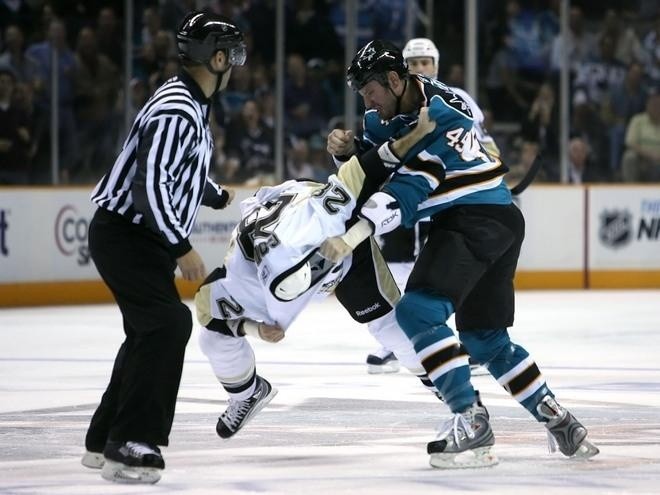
[
  {"x1": 324, "y1": 37, "x2": 587, "y2": 457},
  {"x1": 193, "y1": 107, "x2": 447, "y2": 441},
  {"x1": 365, "y1": 34, "x2": 485, "y2": 368},
  {"x1": 78, "y1": 6, "x2": 250, "y2": 469}
]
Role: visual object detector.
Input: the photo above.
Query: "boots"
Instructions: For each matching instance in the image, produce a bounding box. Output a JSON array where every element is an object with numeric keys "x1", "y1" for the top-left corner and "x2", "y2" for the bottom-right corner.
[
  {"x1": 427, "y1": 390, "x2": 494, "y2": 452},
  {"x1": 537, "y1": 395, "x2": 587, "y2": 457}
]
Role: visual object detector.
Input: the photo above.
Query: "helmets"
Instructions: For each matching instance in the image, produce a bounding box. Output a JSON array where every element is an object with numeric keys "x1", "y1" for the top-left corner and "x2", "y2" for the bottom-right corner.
[
  {"x1": 275, "y1": 262, "x2": 312, "y2": 301},
  {"x1": 177, "y1": 11, "x2": 246, "y2": 65},
  {"x1": 403, "y1": 38, "x2": 440, "y2": 80},
  {"x1": 346, "y1": 39, "x2": 405, "y2": 91}
]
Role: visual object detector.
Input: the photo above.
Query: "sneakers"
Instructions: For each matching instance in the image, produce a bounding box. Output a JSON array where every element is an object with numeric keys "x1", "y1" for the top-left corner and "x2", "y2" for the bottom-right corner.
[
  {"x1": 367, "y1": 347, "x2": 396, "y2": 364},
  {"x1": 85, "y1": 426, "x2": 165, "y2": 468},
  {"x1": 216, "y1": 377, "x2": 272, "y2": 438}
]
